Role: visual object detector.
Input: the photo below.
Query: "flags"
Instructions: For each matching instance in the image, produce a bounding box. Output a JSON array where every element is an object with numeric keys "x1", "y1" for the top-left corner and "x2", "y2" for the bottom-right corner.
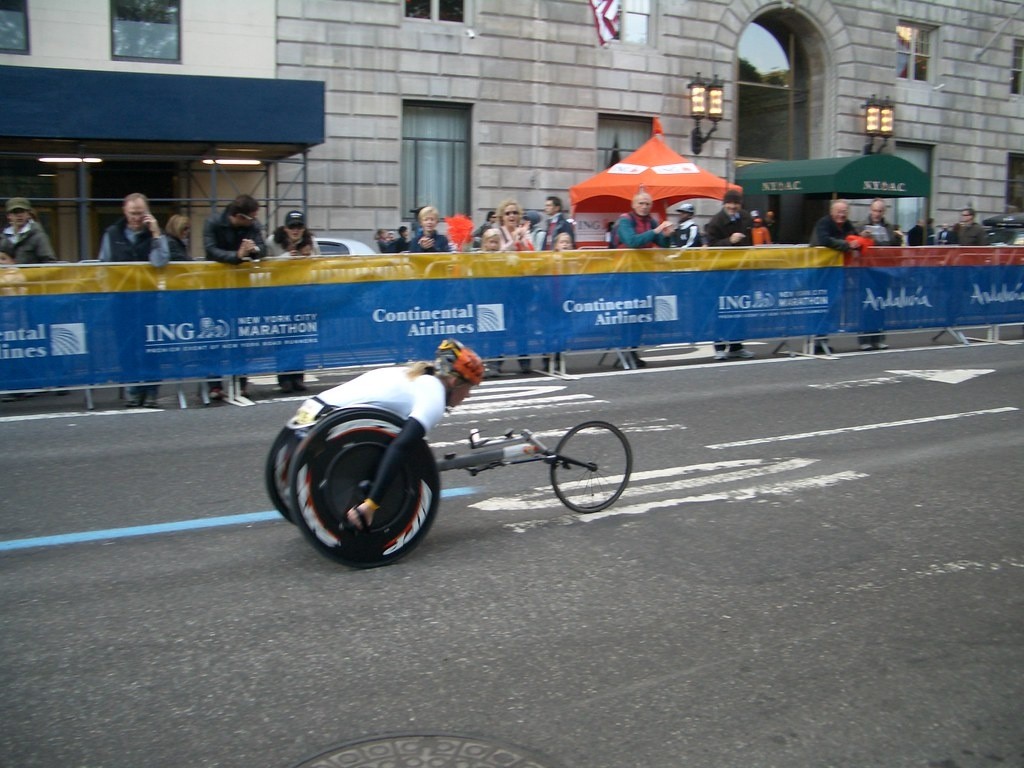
[{"x1": 590, "y1": 0, "x2": 619, "y2": 46}]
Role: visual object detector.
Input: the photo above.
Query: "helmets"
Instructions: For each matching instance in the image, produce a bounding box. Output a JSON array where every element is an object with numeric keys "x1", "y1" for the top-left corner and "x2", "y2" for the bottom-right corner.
[{"x1": 435, "y1": 341, "x2": 484, "y2": 385}]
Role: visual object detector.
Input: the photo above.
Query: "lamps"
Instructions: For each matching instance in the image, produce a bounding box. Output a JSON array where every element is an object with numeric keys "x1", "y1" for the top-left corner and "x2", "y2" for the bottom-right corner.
[
  {"x1": 687, "y1": 72, "x2": 724, "y2": 155},
  {"x1": 859, "y1": 94, "x2": 894, "y2": 156}
]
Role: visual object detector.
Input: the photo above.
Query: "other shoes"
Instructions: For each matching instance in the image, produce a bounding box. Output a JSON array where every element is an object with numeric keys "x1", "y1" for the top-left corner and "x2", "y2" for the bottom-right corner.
[
  {"x1": 126, "y1": 396, "x2": 143, "y2": 407},
  {"x1": 284, "y1": 382, "x2": 294, "y2": 393},
  {"x1": 815, "y1": 346, "x2": 830, "y2": 353},
  {"x1": 860, "y1": 343, "x2": 873, "y2": 350},
  {"x1": 875, "y1": 342, "x2": 888, "y2": 349},
  {"x1": 144, "y1": 396, "x2": 157, "y2": 407},
  {"x1": 295, "y1": 381, "x2": 306, "y2": 391},
  {"x1": 210, "y1": 388, "x2": 221, "y2": 398},
  {"x1": 635, "y1": 357, "x2": 645, "y2": 366},
  {"x1": 520, "y1": 363, "x2": 531, "y2": 373}
]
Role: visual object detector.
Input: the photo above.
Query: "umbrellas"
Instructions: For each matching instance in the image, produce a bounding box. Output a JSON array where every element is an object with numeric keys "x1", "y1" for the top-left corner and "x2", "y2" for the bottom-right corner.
[
  {"x1": 570, "y1": 136, "x2": 742, "y2": 227},
  {"x1": 982, "y1": 214, "x2": 1024, "y2": 228}
]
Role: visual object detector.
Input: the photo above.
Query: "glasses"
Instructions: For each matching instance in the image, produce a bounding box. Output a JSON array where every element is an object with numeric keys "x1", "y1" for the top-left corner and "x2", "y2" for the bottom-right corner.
[
  {"x1": 238, "y1": 214, "x2": 256, "y2": 222},
  {"x1": 288, "y1": 223, "x2": 303, "y2": 230},
  {"x1": 504, "y1": 211, "x2": 518, "y2": 215}
]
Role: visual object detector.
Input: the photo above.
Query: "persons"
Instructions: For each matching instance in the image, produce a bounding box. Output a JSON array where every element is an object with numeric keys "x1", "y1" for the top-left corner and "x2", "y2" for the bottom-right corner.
[
  {"x1": 0, "y1": 200, "x2": 56, "y2": 402},
  {"x1": 284, "y1": 339, "x2": 484, "y2": 528},
  {"x1": 938, "y1": 223, "x2": 960, "y2": 244},
  {"x1": 856, "y1": 198, "x2": 894, "y2": 349},
  {"x1": 675, "y1": 204, "x2": 702, "y2": 247},
  {"x1": 379, "y1": 207, "x2": 449, "y2": 253},
  {"x1": 893, "y1": 225, "x2": 902, "y2": 246},
  {"x1": 201, "y1": 195, "x2": 321, "y2": 397},
  {"x1": 473, "y1": 197, "x2": 576, "y2": 378},
  {"x1": 908, "y1": 218, "x2": 935, "y2": 246},
  {"x1": 748, "y1": 210, "x2": 774, "y2": 245},
  {"x1": 610, "y1": 193, "x2": 674, "y2": 367},
  {"x1": 810, "y1": 200, "x2": 863, "y2": 353},
  {"x1": 958, "y1": 209, "x2": 990, "y2": 246},
  {"x1": 100, "y1": 193, "x2": 192, "y2": 407},
  {"x1": 708, "y1": 190, "x2": 755, "y2": 359}
]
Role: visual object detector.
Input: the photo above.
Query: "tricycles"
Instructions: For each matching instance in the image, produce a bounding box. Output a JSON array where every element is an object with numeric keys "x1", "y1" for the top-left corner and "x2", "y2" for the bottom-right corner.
[{"x1": 265, "y1": 408, "x2": 633, "y2": 569}]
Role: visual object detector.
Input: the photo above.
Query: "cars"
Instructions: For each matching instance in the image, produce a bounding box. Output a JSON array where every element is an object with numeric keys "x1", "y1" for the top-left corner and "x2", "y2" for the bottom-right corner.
[
  {"x1": 314, "y1": 236, "x2": 376, "y2": 256},
  {"x1": 982, "y1": 212, "x2": 1024, "y2": 247}
]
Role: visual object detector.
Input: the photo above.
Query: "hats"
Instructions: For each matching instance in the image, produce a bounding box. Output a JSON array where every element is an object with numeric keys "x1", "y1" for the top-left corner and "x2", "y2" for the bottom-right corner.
[
  {"x1": 285, "y1": 210, "x2": 304, "y2": 227},
  {"x1": 5, "y1": 198, "x2": 30, "y2": 212},
  {"x1": 676, "y1": 203, "x2": 694, "y2": 213},
  {"x1": 723, "y1": 190, "x2": 742, "y2": 205}
]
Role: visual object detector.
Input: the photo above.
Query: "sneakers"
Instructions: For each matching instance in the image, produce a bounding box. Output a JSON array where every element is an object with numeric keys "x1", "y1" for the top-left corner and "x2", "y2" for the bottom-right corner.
[
  {"x1": 714, "y1": 351, "x2": 728, "y2": 359},
  {"x1": 729, "y1": 348, "x2": 754, "y2": 358}
]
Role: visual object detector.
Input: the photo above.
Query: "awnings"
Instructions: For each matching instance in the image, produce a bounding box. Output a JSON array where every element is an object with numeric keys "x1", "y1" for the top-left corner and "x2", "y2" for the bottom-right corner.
[{"x1": 735, "y1": 153, "x2": 930, "y2": 197}]
